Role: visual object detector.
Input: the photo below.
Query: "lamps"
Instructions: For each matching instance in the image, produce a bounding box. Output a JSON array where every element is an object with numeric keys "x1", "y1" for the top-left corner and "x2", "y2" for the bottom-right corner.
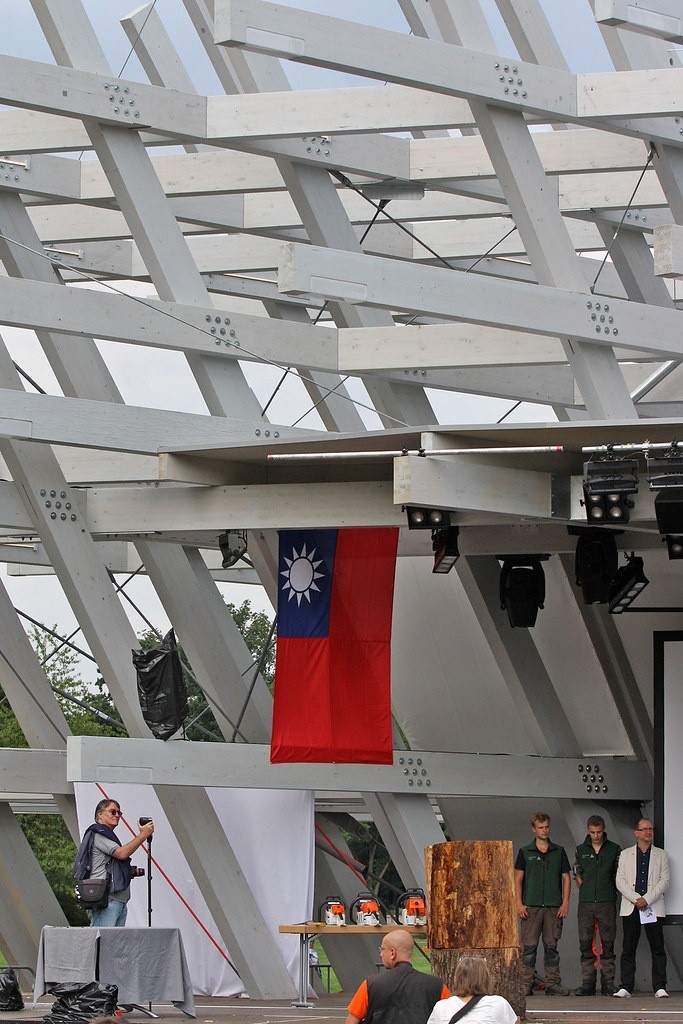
[
  {"x1": 567, "y1": 524, "x2": 649, "y2": 614},
  {"x1": 579, "y1": 444, "x2": 640, "y2": 536},
  {"x1": 219, "y1": 530, "x2": 248, "y2": 569},
  {"x1": 646, "y1": 440, "x2": 683, "y2": 560},
  {"x1": 406, "y1": 506, "x2": 459, "y2": 574},
  {"x1": 494, "y1": 553, "x2": 550, "y2": 629},
  {"x1": 361, "y1": 184, "x2": 427, "y2": 200}
]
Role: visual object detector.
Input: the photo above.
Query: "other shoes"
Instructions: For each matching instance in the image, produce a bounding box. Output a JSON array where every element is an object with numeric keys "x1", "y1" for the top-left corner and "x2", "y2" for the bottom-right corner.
[
  {"x1": 574, "y1": 987, "x2": 597, "y2": 997},
  {"x1": 115, "y1": 1004, "x2": 134, "y2": 1012},
  {"x1": 545, "y1": 984, "x2": 570, "y2": 996},
  {"x1": 600, "y1": 985, "x2": 618, "y2": 995},
  {"x1": 655, "y1": 989, "x2": 669, "y2": 999},
  {"x1": 612, "y1": 989, "x2": 631, "y2": 999},
  {"x1": 523, "y1": 987, "x2": 534, "y2": 997}
]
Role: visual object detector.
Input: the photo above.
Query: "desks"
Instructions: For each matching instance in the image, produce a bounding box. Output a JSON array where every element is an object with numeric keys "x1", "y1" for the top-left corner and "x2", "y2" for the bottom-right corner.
[
  {"x1": 278, "y1": 925, "x2": 427, "y2": 1009},
  {"x1": 32, "y1": 925, "x2": 197, "y2": 1019}
]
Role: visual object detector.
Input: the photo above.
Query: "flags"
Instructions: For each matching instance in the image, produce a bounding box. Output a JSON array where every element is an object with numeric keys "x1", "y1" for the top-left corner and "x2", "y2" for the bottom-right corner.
[{"x1": 270, "y1": 526, "x2": 400, "y2": 766}]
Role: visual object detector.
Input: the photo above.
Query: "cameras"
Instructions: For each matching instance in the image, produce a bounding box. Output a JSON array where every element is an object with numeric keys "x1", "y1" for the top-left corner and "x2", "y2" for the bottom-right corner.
[{"x1": 130, "y1": 866, "x2": 144, "y2": 879}]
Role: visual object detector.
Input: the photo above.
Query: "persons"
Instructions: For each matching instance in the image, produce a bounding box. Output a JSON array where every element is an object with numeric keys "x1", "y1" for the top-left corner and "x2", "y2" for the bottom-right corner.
[
  {"x1": 613, "y1": 818, "x2": 671, "y2": 998},
  {"x1": 574, "y1": 815, "x2": 623, "y2": 997},
  {"x1": 426, "y1": 958, "x2": 519, "y2": 1024},
  {"x1": 514, "y1": 812, "x2": 571, "y2": 996},
  {"x1": 344, "y1": 930, "x2": 450, "y2": 1024},
  {"x1": 77, "y1": 799, "x2": 154, "y2": 925}
]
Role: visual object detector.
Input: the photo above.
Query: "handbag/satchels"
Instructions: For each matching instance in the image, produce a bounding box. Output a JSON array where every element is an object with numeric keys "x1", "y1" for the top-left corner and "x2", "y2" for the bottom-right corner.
[{"x1": 76, "y1": 876, "x2": 110, "y2": 912}]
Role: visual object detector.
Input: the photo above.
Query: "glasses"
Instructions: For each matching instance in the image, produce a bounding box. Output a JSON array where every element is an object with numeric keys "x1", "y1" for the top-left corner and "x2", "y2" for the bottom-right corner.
[
  {"x1": 635, "y1": 826, "x2": 655, "y2": 832},
  {"x1": 378, "y1": 945, "x2": 393, "y2": 954},
  {"x1": 458, "y1": 955, "x2": 487, "y2": 963},
  {"x1": 100, "y1": 809, "x2": 123, "y2": 817}
]
